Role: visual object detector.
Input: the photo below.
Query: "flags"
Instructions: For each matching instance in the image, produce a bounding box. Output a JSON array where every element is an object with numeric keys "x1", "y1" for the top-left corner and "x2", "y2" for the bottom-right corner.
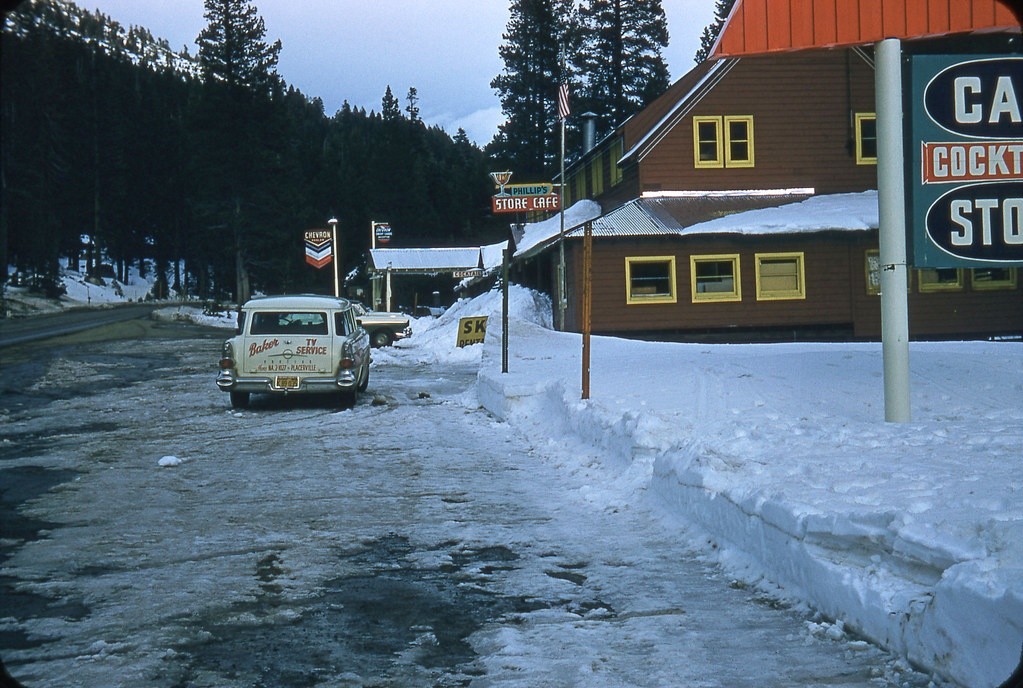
[{"x1": 558, "y1": 41, "x2": 572, "y2": 120}]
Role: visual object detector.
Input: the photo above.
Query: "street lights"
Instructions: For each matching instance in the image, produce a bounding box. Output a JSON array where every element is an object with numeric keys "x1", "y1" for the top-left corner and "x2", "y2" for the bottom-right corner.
[{"x1": 328, "y1": 216, "x2": 339, "y2": 297}]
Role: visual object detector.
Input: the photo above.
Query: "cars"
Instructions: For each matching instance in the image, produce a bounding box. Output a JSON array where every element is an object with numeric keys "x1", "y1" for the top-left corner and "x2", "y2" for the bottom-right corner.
[
  {"x1": 216, "y1": 295, "x2": 371, "y2": 410},
  {"x1": 351, "y1": 301, "x2": 412, "y2": 347}
]
